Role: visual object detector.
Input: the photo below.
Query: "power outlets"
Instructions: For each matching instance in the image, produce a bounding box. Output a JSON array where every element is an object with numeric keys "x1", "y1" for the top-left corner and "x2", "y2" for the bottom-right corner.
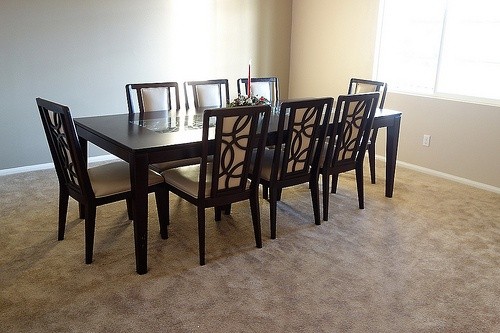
[{"x1": 422, "y1": 134, "x2": 431, "y2": 147}]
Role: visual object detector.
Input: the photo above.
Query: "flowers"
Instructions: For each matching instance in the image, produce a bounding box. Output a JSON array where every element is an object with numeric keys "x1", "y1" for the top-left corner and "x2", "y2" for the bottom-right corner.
[{"x1": 226, "y1": 94, "x2": 271, "y2": 107}]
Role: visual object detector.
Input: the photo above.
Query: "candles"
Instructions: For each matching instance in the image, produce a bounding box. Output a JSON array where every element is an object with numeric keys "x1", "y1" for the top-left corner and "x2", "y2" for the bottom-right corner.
[{"x1": 247, "y1": 59, "x2": 252, "y2": 98}]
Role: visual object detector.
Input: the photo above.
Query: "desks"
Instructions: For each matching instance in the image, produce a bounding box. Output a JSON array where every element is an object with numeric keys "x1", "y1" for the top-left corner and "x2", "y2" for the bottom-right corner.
[{"x1": 71, "y1": 105, "x2": 402, "y2": 275}]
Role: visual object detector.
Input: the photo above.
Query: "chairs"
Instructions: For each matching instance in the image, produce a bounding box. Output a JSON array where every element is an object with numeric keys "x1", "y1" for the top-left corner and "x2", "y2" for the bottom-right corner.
[
  {"x1": 122, "y1": 75, "x2": 279, "y2": 173},
  {"x1": 35, "y1": 97, "x2": 169, "y2": 265},
  {"x1": 158, "y1": 103, "x2": 271, "y2": 267},
  {"x1": 311, "y1": 92, "x2": 380, "y2": 221},
  {"x1": 245, "y1": 97, "x2": 335, "y2": 240},
  {"x1": 333, "y1": 79, "x2": 387, "y2": 184}
]
